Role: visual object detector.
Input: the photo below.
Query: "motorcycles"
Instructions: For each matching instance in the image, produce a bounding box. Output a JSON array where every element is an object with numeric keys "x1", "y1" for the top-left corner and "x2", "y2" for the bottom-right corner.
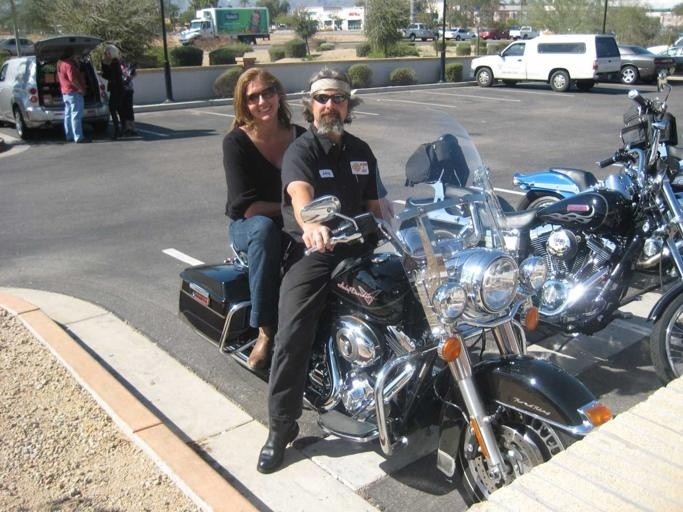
[
  {"x1": 503, "y1": 121, "x2": 682, "y2": 277},
  {"x1": 385, "y1": 86, "x2": 682, "y2": 387},
  {"x1": 163, "y1": 101, "x2": 616, "y2": 512}
]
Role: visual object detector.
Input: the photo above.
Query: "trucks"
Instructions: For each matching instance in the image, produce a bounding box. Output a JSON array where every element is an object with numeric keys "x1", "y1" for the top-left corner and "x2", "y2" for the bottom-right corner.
[
  {"x1": 468, "y1": 31, "x2": 623, "y2": 93},
  {"x1": 177, "y1": 7, "x2": 273, "y2": 48}
]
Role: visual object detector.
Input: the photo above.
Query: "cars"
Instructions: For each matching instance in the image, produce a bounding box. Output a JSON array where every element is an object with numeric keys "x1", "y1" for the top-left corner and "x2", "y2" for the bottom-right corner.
[
  {"x1": 613, "y1": 44, "x2": 664, "y2": 85},
  {"x1": 654, "y1": 46, "x2": 682, "y2": 85},
  {"x1": 396, "y1": 21, "x2": 541, "y2": 44},
  {"x1": 0, "y1": 34, "x2": 110, "y2": 140},
  {"x1": 668, "y1": 36, "x2": 682, "y2": 47},
  {"x1": 0, "y1": 37, "x2": 35, "y2": 56}
]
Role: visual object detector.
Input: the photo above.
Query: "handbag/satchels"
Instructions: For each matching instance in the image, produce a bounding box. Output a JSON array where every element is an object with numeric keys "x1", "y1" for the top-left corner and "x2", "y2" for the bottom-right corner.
[
  {"x1": 405, "y1": 139, "x2": 469, "y2": 188},
  {"x1": 621, "y1": 104, "x2": 677, "y2": 147}
]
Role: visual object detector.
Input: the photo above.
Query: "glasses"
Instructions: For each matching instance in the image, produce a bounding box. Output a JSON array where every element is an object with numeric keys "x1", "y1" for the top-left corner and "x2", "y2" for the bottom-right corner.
[
  {"x1": 313, "y1": 94, "x2": 346, "y2": 103},
  {"x1": 248, "y1": 86, "x2": 276, "y2": 104}
]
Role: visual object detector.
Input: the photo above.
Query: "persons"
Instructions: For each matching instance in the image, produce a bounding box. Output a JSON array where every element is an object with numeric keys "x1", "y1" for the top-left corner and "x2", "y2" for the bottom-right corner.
[
  {"x1": 258, "y1": 66, "x2": 396, "y2": 474},
  {"x1": 114, "y1": 46, "x2": 137, "y2": 132},
  {"x1": 56, "y1": 47, "x2": 91, "y2": 143},
  {"x1": 98, "y1": 43, "x2": 128, "y2": 136},
  {"x1": 222, "y1": 66, "x2": 307, "y2": 373}
]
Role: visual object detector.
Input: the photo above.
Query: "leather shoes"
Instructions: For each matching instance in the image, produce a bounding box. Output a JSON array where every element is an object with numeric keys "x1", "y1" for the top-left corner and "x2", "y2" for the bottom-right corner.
[
  {"x1": 257, "y1": 423, "x2": 298, "y2": 475},
  {"x1": 247, "y1": 328, "x2": 274, "y2": 370}
]
